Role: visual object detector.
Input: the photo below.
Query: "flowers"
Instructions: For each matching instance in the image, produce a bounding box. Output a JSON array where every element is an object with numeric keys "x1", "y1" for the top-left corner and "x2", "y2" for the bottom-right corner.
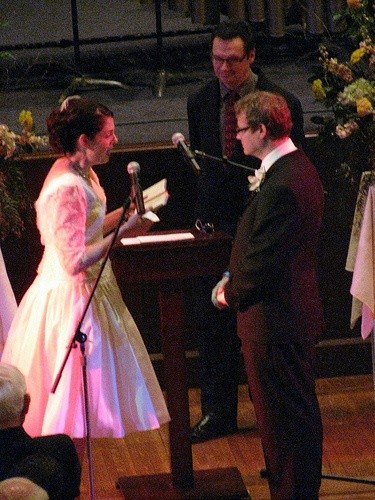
[
  {"x1": 290, "y1": 0, "x2": 375, "y2": 172},
  {"x1": 0, "y1": 107, "x2": 50, "y2": 241}
]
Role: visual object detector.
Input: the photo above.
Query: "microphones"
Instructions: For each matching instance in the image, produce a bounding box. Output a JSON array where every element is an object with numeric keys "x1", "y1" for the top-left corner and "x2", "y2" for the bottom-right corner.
[
  {"x1": 127, "y1": 161, "x2": 146, "y2": 215},
  {"x1": 172, "y1": 131, "x2": 201, "y2": 174}
]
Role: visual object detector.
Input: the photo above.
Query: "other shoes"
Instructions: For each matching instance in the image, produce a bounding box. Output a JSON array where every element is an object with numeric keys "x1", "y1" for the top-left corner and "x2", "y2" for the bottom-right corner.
[{"x1": 261, "y1": 468, "x2": 268, "y2": 478}]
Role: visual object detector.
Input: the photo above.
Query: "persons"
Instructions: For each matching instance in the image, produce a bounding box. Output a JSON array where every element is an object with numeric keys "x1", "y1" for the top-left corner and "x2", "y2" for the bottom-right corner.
[
  {"x1": 0, "y1": 364, "x2": 82, "y2": 500},
  {"x1": 0, "y1": 477, "x2": 49, "y2": 500},
  {"x1": 187, "y1": 23, "x2": 304, "y2": 438},
  {"x1": 212, "y1": 91, "x2": 325, "y2": 500},
  {"x1": 0, "y1": 97, "x2": 171, "y2": 459}
]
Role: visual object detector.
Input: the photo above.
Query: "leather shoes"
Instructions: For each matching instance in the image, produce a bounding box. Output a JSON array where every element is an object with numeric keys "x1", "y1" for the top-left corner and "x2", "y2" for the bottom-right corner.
[{"x1": 190, "y1": 413, "x2": 239, "y2": 444}]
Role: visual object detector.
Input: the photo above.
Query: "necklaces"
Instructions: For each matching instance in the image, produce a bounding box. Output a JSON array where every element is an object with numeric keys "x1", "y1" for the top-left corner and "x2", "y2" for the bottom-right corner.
[{"x1": 74, "y1": 164, "x2": 88, "y2": 179}]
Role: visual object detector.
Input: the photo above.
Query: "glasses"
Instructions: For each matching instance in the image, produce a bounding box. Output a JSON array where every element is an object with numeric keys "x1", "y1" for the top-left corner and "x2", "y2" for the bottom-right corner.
[
  {"x1": 233, "y1": 123, "x2": 252, "y2": 134},
  {"x1": 209, "y1": 49, "x2": 249, "y2": 64}
]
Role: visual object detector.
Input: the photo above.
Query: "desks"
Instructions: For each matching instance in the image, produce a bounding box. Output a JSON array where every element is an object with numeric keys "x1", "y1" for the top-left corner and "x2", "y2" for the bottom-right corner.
[{"x1": 108, "y1": 230, "x2": 233, "y2": 500}]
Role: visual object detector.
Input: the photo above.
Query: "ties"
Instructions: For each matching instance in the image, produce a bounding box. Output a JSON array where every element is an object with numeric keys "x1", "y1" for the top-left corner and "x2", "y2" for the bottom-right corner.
[{"x1": 222, "y1": 91, "x2": 239, "y2": 159}]
